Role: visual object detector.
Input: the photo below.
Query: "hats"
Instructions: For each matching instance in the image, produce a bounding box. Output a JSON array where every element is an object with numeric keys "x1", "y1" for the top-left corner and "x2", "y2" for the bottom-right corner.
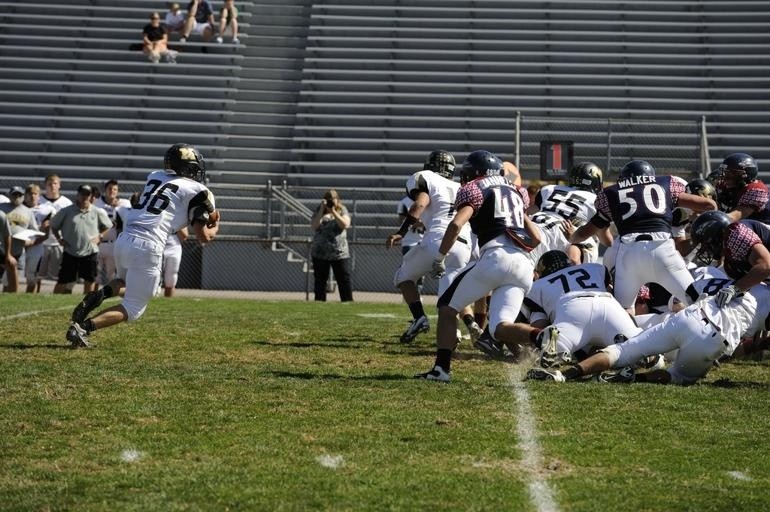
[
  {"x1": 79, "y1": 185, "x2": 93, "y2": 192},
  {"x1": 10, "y1": 186, "x2": 25, "y2": 195}
]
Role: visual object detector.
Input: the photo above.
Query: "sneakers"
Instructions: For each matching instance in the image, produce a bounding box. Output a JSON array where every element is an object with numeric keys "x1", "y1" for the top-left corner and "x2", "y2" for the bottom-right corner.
[
  {"x1": 399, "y1": 315, "x2": 430, "y2": 344},
  {"x1": 413, "y1": 365, "x2": 451, "y2": 383},
  {"x1": 66, "y1": 291, "x2": 99, "y2": 350},
  {"x1": 215, "y1": 37, "x2": 239, "y2": 44},
  {"x1": 468, "y1": 321, "x2": 524, "y2": 364},
  {"x1": 524, "y1": 326, "x2": 660, "y2": 384},
  {"x1": 176, "y1": 35, "x2": 187, "y2": 42},
  {"x1": 450, "y1": 328, "x2": 462, "y2": 352}
]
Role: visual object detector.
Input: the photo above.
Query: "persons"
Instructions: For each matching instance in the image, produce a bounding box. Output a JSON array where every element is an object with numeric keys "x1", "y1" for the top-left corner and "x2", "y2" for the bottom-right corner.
[
  {"x1": 383, "y1": 148, "x2": 770, "y2": 385},
  {"x1": 0, "y1": 173, "x2": 190, "y2": 299},
  {"x1": 141, "y1": 0, "x2": 240, "y2": 64},
  {"x1": 64, "y1": 142, "x2": 220, "y2": 349},
  {"x1": 309, "y1": 188, "x2": 353, "y2": 303}
]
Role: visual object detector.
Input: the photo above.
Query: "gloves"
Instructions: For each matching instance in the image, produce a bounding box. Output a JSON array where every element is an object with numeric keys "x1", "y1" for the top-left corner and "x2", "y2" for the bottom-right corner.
[
  {"x1": 715, "y1": 285, "x2": 751, "y2": 308},
  {"x1": 431, "y1": 257, "x2": 446, "y2": 279}
]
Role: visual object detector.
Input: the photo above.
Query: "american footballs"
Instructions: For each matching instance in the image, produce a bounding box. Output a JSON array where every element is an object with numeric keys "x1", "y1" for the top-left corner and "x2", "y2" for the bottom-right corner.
[{"x1": 209, "y1": 211, "x2": 217, "y2": 224}]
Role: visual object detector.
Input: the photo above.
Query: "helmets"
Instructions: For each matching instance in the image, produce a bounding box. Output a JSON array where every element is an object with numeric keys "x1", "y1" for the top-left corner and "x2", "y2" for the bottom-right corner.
[
  {"x1": 619, "y1": 160, "x2": 656, "y2": 181},
  {"x1": 165, "y1": 143, "x2": 206, "y2": 182},
  {"x1": 567, "y1": 162, "x2": 603, "y2": 193},
  {"x1": 424, "y1": 149, "x2": 504, "y2": 186},
  {"x1": 691, "y1": 210, "x2": 736, "y2": 265},
  {"x1": 684, "y1": 153, "x2": 759, "y2": 203},
  {"x1": 536, "y1": 249, "x2": 577, "y2": 279}
]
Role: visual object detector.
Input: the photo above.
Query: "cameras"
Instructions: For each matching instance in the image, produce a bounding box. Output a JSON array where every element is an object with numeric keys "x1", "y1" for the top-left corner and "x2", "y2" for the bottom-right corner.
[{"x1": 325, "y1": 199, "x2": 334, "y2": 208}]
